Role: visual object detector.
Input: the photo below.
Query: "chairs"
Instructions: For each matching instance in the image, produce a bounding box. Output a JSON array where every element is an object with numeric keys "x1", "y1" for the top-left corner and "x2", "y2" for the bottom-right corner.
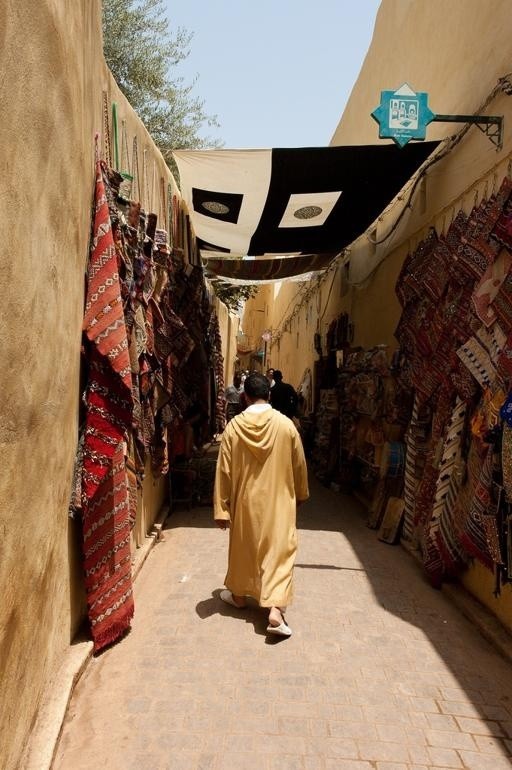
[{"x1": 166, "y1": 472, "x2": 192, "y2": 512}]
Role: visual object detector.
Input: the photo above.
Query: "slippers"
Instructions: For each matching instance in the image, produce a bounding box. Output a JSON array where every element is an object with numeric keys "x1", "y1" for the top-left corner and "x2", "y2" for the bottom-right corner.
[
  {"x1": 219, "y1": 589, "x2": 242, "y2": 608},
  {"x1": 267, "y1": 620, "x2": 292, "y2": 636}
]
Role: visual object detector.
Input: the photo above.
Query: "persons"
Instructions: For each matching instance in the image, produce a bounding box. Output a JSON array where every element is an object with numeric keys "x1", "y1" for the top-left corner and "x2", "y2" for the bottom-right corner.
[
  {"x1": 222, "y1": 367, "x2": 300, "y2": 426},
  {"x1": 211, "y1": 370, "x2": 310, "y2": 638}
]
